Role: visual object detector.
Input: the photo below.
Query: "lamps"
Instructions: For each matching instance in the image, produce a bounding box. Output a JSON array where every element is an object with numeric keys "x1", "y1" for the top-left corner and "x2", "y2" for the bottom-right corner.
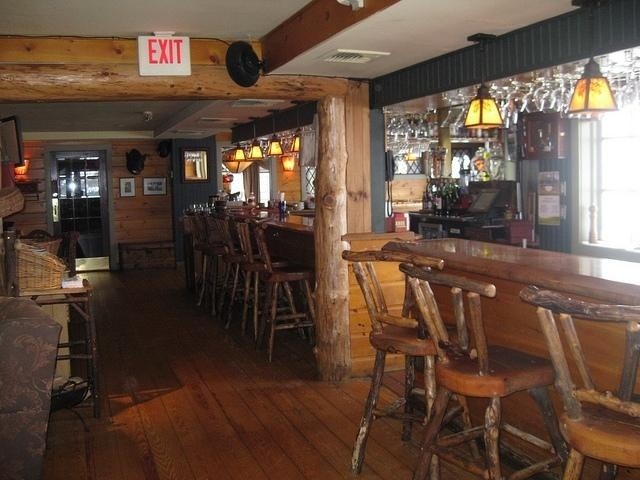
[
  {"x1": 13, "y1": 159, "x2": 31, "y2": 181},
  {"x1": 465, "y1": 33, "x2": 505, "y2": 129},
  {"x1": 569, "y1": 0, "x2": 619, "y2": 112},
  {"x1": 221, "y1": 100, "x2": 304, "y2": 173},
  {"x1": 184, "y1": 161, "x2": 197, "y2": 177}
]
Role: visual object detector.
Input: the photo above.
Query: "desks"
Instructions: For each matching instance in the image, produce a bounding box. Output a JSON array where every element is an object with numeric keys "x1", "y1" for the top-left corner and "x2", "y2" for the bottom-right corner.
[
  {"x1": 12, "y1": 278, "x2": 101, "y2": 420},
  {"x1": 217, "y1": 206, "x2": 317, "y2": 278},
  {"x1": 386, "y1": 235, "x2": 640, "y2": 457}
]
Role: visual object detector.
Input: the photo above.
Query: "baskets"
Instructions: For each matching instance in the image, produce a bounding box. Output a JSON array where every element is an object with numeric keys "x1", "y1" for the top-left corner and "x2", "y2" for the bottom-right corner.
[
  {"x1": 0, "y1": 185, "x2": 25, "y2": 218},
  {"x1": 14, "y1": 242, "x2": 67, "y2": 292},
  {"x1": 20, "y1": 229, "x2": 63, "y2": 257}
]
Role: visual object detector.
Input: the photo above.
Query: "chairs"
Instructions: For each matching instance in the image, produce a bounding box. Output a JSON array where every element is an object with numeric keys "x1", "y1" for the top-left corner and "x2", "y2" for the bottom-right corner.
[
  {"x1": 520, "y1": 279, "x2": 640, "y2": 480},
  {"x1": 246, "y1": 219, "x2": 320, "y2": 362},
  {"x1": 397, "y1": 259, "x2": 566, "y2": 479},
  {"x1": 181, "y1": 210, "x2": 246, "y2": 317},
  {"x1": 225, "y1": 215, "x2": 286, "y2": 334},
  {"x1": 340, "y1": 244, "x2": 482, "y2": 480}
]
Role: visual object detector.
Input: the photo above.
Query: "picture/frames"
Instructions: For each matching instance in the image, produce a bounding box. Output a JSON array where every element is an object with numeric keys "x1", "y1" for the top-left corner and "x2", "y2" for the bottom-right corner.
[
  {"x1": 119, "y1": 177, "x2": 136, "y2": 197},
  {"x1": 143, "y1": 176, "x2": 168, "y2": 196}
]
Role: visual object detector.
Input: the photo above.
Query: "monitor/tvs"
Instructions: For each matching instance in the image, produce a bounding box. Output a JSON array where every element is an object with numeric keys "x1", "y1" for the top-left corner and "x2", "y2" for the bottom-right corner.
[{"x1": 466, "y1": 189, "x2": 502, "y2": 213}]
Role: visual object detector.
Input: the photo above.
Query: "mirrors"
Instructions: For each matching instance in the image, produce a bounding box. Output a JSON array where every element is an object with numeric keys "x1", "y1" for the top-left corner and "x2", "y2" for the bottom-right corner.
[{"x1": 179, "y1": 146, "x2": 210, "y2": 184}]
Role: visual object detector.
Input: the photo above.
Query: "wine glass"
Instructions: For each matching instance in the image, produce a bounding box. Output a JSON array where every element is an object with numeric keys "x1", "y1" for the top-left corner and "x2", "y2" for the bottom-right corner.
[{"x1": 441, "y1": 48, "x2": 639, "y2": 127}]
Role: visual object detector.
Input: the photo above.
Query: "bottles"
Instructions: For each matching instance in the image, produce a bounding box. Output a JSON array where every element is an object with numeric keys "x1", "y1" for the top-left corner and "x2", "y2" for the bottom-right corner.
[
  {"x1": 423, "y1": 179, "x2": 458, "y2": 214},
  {"x1": 460, "y1": 169, "x2": 471, "y2": 195},
  {"x1": 248, "y1": 193, "x2": 256, "y2": 209}
]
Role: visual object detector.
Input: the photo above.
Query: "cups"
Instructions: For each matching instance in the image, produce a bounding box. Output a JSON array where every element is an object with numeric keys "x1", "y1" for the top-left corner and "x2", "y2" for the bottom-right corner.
[{"x1": 385, "y1": 112, "x2": 429, "y2": 141}]
